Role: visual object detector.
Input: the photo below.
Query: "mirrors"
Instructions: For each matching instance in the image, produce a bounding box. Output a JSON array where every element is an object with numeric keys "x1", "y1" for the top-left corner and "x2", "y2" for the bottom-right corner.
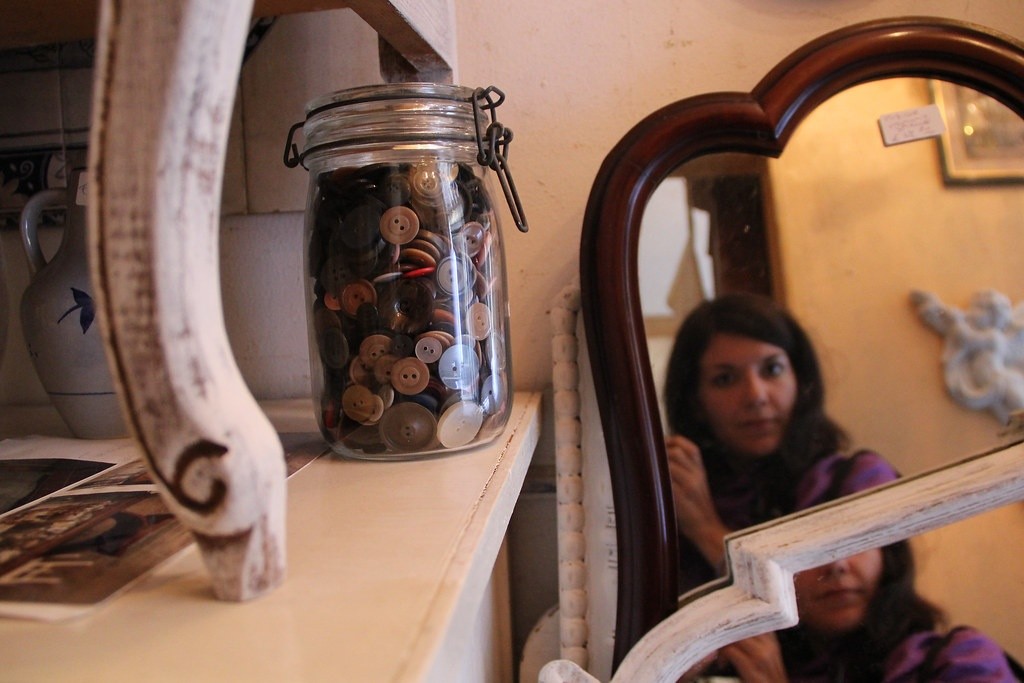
[{"x1": 576, "y1": 19, "x2": 1024, "y2": 683}]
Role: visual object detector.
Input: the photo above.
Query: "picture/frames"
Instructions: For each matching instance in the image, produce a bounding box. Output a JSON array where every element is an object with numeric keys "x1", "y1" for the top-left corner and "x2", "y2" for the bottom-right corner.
[{"x1": 926, "y1": 78, "x2": 1024, "y2": 184}]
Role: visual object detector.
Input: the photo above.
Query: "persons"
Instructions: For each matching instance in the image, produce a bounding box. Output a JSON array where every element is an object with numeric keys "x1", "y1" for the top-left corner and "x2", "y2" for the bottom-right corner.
[
  {"x1": 665, "y1": 298, "x2": 897, "y2": 584},
  {"x1": 681, "y1": 449, "x2": 1023, "y2": 683}
]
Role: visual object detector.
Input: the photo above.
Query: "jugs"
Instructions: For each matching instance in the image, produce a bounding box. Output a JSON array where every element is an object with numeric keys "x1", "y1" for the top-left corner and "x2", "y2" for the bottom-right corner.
[{"x1": 20, "y1": 164, "x2": 132, "y2": 438}]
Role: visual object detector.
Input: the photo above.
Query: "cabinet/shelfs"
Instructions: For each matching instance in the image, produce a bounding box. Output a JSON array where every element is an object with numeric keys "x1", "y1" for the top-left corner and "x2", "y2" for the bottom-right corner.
[{"x1": 0, "y1": 388, "x2": 544, "y2": 683}]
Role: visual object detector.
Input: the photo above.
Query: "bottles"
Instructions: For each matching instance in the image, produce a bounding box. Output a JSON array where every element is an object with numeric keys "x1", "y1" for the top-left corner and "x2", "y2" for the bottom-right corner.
[{"x1": 304, "y1": 84, "x2": 515, "y2": 460}]
